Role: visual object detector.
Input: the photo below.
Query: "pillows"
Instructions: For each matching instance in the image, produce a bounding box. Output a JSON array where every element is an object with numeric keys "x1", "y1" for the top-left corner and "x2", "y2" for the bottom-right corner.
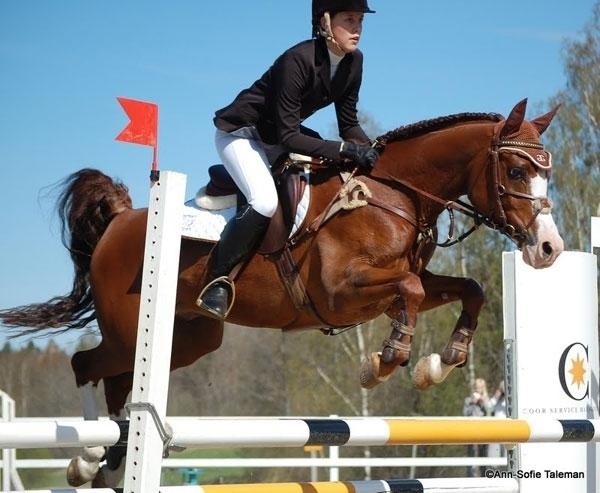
[{"x1": 312, "y1": 0, "x2": 374, "y2": 23}]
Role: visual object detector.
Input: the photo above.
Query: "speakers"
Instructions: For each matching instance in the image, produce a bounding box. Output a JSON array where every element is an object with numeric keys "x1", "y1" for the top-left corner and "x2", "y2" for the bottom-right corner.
[{"x1": 199, "y1": 204, "x2": 271, "y2": 318}]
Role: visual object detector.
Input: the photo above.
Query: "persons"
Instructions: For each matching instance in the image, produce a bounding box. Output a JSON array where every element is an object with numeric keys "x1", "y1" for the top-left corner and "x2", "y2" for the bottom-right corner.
[
  {"x1": 464, "y1": 377, "x2": 508, "y2": 477},
  {"x1": 196, "y1": 0, "x2": 381, "y2": 323}
]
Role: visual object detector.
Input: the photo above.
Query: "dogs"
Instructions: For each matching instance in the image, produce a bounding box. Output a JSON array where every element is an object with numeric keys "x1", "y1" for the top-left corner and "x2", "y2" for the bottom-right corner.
[{"x1": 0, "y1": 96, "x2": 565, "y2": 487}]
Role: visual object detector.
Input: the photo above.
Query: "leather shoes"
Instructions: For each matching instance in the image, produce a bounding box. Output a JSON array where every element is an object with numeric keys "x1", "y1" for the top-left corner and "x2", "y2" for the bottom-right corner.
[{"x1": 340, "y1": 142, "x2": 379, "y2": 173}]
